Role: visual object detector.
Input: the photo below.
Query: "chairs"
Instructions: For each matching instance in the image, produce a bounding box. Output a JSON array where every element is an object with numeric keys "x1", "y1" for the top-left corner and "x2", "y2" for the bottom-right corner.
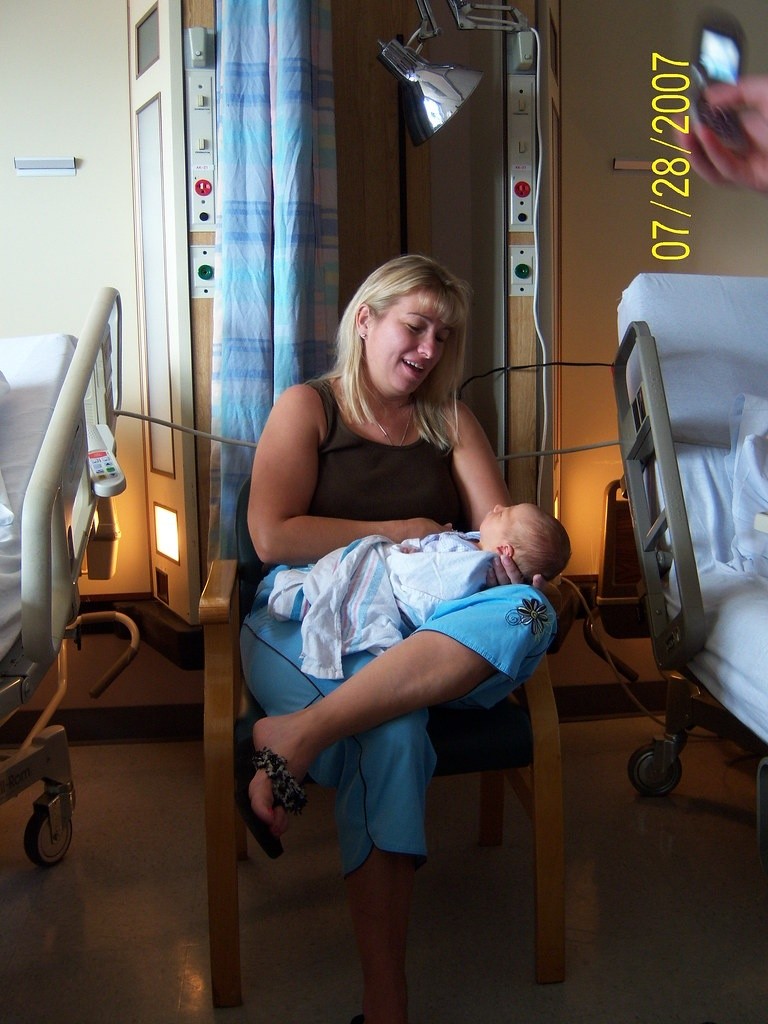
[{"x1": 197, "y1": 473, "x2": 570, "y2": 1008}]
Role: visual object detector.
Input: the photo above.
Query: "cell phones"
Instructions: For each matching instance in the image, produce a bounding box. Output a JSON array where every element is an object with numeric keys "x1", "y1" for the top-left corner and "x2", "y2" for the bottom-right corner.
[{"x1": 684, "y1": 10, "x2": 750, "y2": 152}]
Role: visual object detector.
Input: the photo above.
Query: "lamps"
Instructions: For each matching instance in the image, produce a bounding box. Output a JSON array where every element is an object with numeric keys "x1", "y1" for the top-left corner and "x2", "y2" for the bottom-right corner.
[{"x1": 375, "y1": 31, "x2": 486, "y2": 148}]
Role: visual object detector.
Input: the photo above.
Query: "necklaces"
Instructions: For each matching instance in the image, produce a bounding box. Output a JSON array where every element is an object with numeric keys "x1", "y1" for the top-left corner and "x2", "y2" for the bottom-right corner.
[{"x1": 376, "y1": 404, "x2": 413, "y2": 447}]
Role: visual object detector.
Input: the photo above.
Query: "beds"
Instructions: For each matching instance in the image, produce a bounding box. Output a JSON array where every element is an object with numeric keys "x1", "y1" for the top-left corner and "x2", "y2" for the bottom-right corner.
[
  {"x1": 609, "y1": 272, "x2": 768, "y2": 874},
  {"x1": 0, "y1": 287, "x2": 144, "y2": 868}
]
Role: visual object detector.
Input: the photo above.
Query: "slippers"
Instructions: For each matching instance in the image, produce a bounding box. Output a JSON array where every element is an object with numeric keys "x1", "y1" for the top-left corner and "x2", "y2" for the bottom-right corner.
[{"x1": 233, "y1": 716, "x2": 302, "y2": 860}]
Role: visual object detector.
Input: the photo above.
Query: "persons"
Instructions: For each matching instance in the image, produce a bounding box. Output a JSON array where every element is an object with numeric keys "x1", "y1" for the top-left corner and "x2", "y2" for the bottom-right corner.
[
  {"x1": 240, "y1": 254, "x2": 561, "y2": 1024},
  {"x1": 267, "y1": 502, "x2": 572, "y2": 680}
]
